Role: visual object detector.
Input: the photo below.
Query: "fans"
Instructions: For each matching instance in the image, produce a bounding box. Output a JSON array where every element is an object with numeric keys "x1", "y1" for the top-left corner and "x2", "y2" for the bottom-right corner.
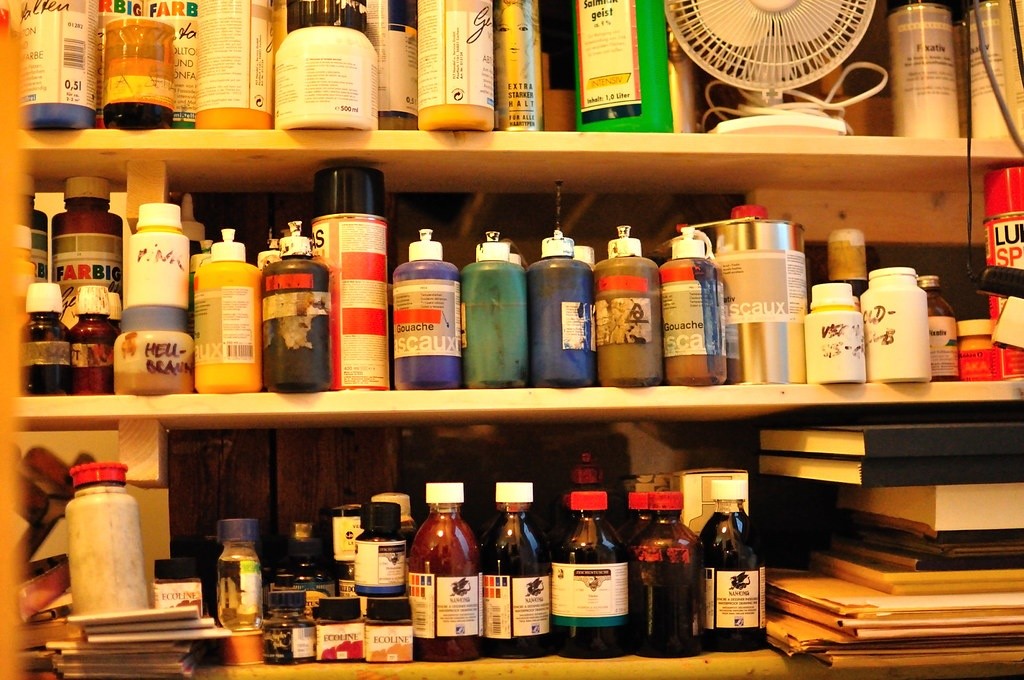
[{"x1": 662, "y1": 0, "x2": 889, "y2": 134}]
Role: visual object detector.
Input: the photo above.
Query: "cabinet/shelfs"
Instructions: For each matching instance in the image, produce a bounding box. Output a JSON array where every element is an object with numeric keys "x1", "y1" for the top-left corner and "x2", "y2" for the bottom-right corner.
[{"x1": 0, "y1": 0, "x2": 1023, "y2": 680}]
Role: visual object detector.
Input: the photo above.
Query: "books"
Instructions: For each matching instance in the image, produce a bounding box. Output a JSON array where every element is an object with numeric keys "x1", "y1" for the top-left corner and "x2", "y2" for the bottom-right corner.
[
  {"x1": 14, "y1": 592, "x2": 233, "y2": 680},
  {"x1": 754, "y1": 421, "x2": 1024, "y2": 666}
]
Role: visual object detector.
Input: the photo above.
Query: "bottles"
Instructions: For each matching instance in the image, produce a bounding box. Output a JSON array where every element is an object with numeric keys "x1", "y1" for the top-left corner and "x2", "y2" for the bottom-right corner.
[
  {"x1": 887, "y1": 0, "x2": 1024, "y2": 144},
  {"x1": 218, "y1": 478, "x2": 765, "y2": 665},
  {"x1": 8, "y1": 0, "x2": 694, "y2": 134},
  {"x1": 11, "y1": 164, "x2": 994, "y2": 393},
  {"x1": 61, "y1": 463, "x2": 145, "y2": 618}
]
